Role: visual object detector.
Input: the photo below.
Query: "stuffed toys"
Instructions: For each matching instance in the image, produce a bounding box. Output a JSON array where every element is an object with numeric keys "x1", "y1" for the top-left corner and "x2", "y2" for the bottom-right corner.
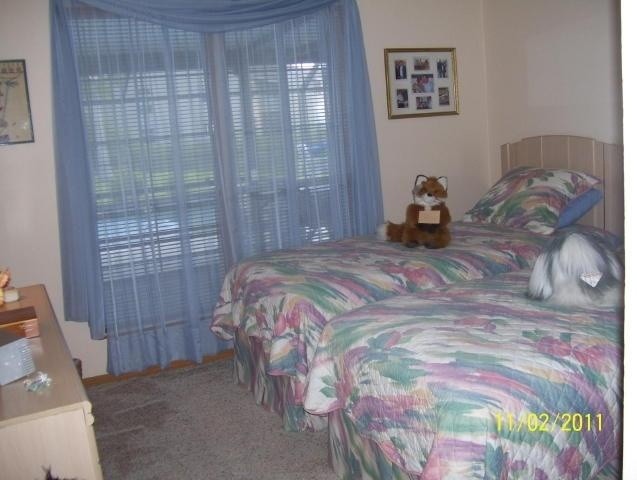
[{"x1": 372, "y1": 174, "x2": 453, "y2": 252}]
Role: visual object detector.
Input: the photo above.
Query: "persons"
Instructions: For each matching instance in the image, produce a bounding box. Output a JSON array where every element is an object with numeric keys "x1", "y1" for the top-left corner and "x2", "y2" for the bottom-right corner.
[{"x1": 394, "y1": 59, "x2": 450, "y2": 111}]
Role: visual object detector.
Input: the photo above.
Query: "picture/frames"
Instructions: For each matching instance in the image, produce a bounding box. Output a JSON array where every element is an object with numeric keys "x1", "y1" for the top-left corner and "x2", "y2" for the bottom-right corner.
[
  {"x1": 382, "y1": 47, "x2": 460, "y2": 120},
  {"x1": 0, "y1": 59, "x2": 34, "y2": 142}
]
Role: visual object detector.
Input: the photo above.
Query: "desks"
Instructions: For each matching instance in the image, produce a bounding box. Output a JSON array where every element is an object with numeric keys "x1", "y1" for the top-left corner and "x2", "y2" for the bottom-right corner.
[{"x1": 1, "y1": 284, "x2": 103, "y2": 478}]
